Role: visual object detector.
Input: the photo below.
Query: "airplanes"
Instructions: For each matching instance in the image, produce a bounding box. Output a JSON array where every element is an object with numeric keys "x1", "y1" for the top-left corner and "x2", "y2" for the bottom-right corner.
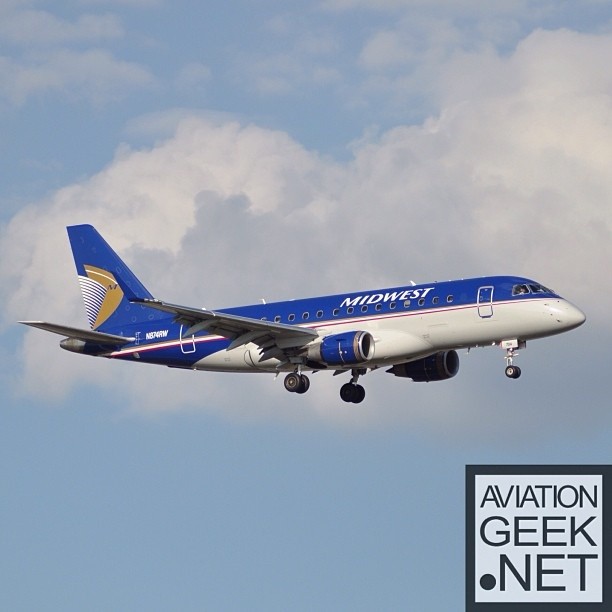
[{"x1": 19, "y1": 225, "x2": 584, "y2": 401}]
